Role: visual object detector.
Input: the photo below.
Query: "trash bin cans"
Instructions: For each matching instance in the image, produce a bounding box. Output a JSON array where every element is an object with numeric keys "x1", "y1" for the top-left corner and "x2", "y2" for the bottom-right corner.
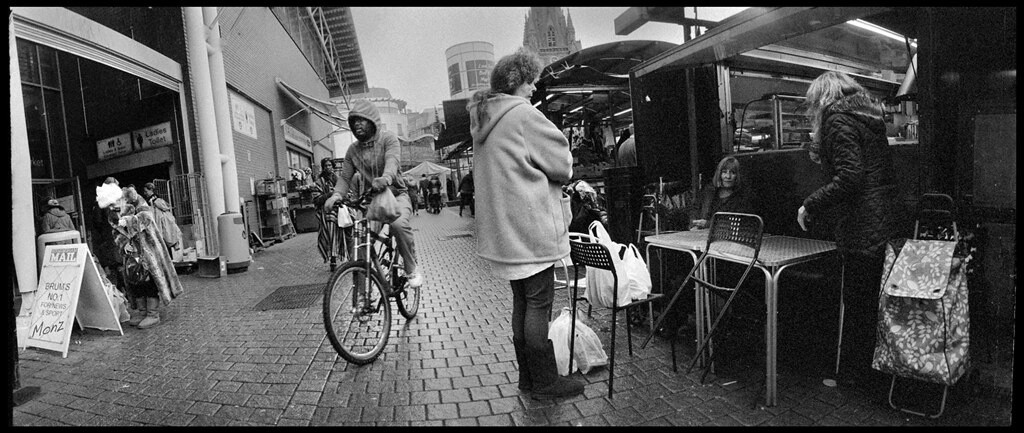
[
  {"x1": 218, "y1": 210, "x2": 251, "y2": 271},
  {"x1": 38, "y1": 231, "x2": 82, "y2": 274}
]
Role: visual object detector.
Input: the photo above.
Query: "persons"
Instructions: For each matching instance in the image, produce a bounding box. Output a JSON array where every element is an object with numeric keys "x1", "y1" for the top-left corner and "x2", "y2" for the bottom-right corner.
[
  {"x1": 321, "y1": 99, "x2": 422, "y2": 314},
  {"x1": 616, "y1": 122, "x2": 637, "y2": 168},
  {"x1": 41, "y1": 198, "x2": 78, "y2": 246},
  {"x1": 402, "y1": 177, "x2": 409, "y2": 189},
  {"x1": 418, "y1": 173, "x2": 431, "y2": 211},
  {"x1": 794, "y1": 69, "x2": 906, "y2": 389},
  {"x1": 458, "y1": 170, "x2": 476, "y2": 218},
  {"x1": 427, "y1": 174, "x2": 442, "y2": 214},
  {"x1": 313, "y1": 154, "x2": 357, "y2": 264},
  {"x1": 99, "y1": 179, "x2": 183, "y2": 331},
  {"x1": 408, "y1": 176, "x2": 420, "y2": 216},
  {"x1": 446, "y1": 178, "x2": 457, "y2": 202},
  {"x1": 141, "y1": 183, "x2": 173, "y2": 260},
  {"x1": 680, "y1": 154, "x2": 752, "y2": 233},
  {"x1": 468, "y1": 47, "x2": 585, "y2": 402}
]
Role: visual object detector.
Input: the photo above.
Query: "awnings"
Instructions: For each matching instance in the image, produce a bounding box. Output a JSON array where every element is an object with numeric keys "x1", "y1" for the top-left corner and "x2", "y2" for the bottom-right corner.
[{"x1": 276, "y1": 76, "x2": 356, "y2": 137}]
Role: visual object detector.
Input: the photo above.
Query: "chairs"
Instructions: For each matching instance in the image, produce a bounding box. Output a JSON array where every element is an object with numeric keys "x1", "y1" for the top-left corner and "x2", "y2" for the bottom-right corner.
[
  {"x1": 640, "y1": 211, "x2": 769, "y2": 406},
  {"x1": 566, "y1": 238, "x2": 678, "y2": 399}
]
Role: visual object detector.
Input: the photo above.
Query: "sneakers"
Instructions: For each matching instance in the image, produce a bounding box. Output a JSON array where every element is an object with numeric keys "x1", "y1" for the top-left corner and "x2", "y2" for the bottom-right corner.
[{"x1": 405, "y1": 266, "x2": 422, "y2": 289}]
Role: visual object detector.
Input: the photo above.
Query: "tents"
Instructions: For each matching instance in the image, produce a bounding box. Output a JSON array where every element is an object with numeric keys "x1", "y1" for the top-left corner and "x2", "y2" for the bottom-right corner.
[{"x1": 400, "y1": 160, "x2": 460, "y2": 195}]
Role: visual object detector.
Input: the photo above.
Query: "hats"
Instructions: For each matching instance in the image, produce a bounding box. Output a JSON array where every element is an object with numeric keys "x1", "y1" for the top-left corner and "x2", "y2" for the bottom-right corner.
[{"x1": 47, "y1": 199, "x2": 59, "y2": 206}]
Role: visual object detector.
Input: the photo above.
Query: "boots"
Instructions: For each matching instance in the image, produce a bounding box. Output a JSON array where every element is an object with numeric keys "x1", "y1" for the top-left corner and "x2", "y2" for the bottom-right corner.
[
  {"x1": 129, "y1": 295, "x2": 161, "y2": 329},
  {"x1": 512, "y1": 335, "x2": 584, "y2": 401}
]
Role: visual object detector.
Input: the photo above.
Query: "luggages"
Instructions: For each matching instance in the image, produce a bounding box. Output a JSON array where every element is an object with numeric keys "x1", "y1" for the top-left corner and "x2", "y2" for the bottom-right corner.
[{"x1": 867, "y1": 191, "x2": 981, "y2": 420}]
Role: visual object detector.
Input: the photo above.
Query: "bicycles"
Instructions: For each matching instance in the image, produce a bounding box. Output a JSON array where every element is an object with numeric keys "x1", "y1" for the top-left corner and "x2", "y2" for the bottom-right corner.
[{"x1": 322, "y1": 185, "x2": 420, "y2": 366}]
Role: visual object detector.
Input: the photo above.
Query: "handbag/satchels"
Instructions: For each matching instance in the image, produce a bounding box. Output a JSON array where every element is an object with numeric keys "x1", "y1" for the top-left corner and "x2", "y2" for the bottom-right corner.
[
  {"x1": 366, "y1": 187, "x2": 403, "y2": 224},
  {"x1": 584, "y1": 219, "x2": 653, "y2": 308},
  {"x1": 125, "y1": 252, "x2": 152, "y2": 290},
  {"x1": 545, "y1": 305, "x2": 608, "y2": 377}
]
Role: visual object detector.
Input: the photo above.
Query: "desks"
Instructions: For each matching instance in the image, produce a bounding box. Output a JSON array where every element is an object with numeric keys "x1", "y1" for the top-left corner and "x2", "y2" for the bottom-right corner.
[{"x1": 644, "y1": 224, "x2": 847, "y2": 409}]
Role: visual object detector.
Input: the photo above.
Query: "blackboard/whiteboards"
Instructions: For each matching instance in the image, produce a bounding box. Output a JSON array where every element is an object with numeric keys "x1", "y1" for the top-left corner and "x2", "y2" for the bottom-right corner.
[{"x1": 28, "y1": 242, "x2": 121, "y2": 352}]
